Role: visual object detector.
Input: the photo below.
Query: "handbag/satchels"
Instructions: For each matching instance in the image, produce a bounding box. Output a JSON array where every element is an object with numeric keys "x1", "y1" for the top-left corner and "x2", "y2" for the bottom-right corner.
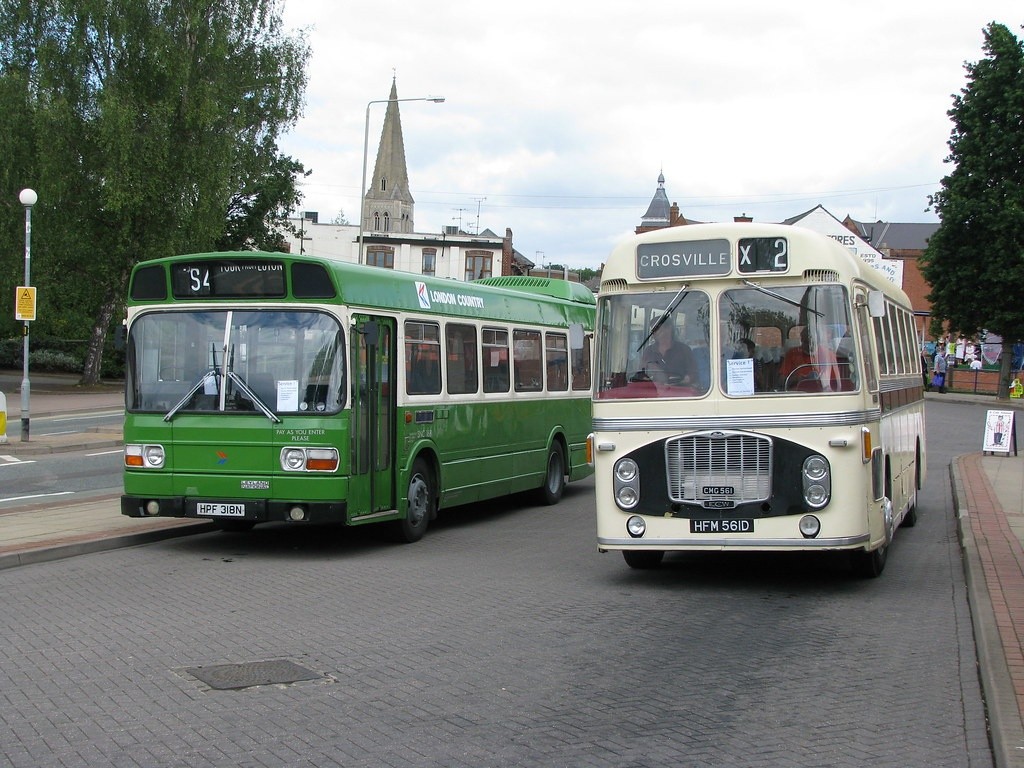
[{"x1": 932, "y1": 374, "x2": 943, "y2": 387}]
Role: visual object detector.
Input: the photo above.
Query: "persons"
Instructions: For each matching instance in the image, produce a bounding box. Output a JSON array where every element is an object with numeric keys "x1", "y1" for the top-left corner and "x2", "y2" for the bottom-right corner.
[
  {"x1": 824, "y1": 327, "x2": 838, "y2": 339},
  {"x1": 920, "y1": 351, "x2": 929, "y2": 391},
  {"x1": 930, "y1": 345, "x2": 946, "y2": 395},
  {"x1": 779, "y1": 327, "x2": 840, "y2": 392},
  {"x1": 736, "y1": 339, "x2": 763, "y2": 391},
  {"x1": 993, "y1": 416, "x2": 1005, "y2": 446},
  {"x1": 639, "y1": 316, "x2": 696, "y2": 386},
  {"x1": 956, "y1": 334, "x2": 975, "y2": 366}
]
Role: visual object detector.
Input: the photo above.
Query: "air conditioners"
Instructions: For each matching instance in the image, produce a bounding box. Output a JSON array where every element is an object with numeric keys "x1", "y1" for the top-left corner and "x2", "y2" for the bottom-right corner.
[
  {"x1": 442, "y1": 225, "x2": 458, "y2": 235},
  {"x1": 301, "y1": 211, "x2": 319, "y2": 224}
]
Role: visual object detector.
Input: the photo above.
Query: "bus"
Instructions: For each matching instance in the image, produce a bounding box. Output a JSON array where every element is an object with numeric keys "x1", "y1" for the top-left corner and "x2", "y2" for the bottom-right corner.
[
  {"x1": 120, "y1": 250, "x2": 597, "y2": 544},
  {"x1": 585, "y1": 222, "x2": 928, "y2": 576}
]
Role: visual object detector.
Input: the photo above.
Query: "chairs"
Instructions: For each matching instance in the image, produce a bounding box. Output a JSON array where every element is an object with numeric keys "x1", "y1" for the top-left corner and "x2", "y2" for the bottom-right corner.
[
  {"x1": 243, "y1": 374, "x2": 275, "y2": 392},
  {"x1": 417, "y1": 359, "x2": 587, "y2": 392},
  {"x1": 690, "y1": 336, "x2": 890, "y2": 391}
]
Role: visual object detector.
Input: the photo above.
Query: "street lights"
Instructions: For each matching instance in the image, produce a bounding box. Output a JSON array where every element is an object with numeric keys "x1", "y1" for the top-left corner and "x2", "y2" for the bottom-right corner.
[
  {"x1": 18, "y1": 186, "x2": 38, "y2": 443},
  {"x1": 358, "y1": 96, "x2": 446, "y2": 266}
]
